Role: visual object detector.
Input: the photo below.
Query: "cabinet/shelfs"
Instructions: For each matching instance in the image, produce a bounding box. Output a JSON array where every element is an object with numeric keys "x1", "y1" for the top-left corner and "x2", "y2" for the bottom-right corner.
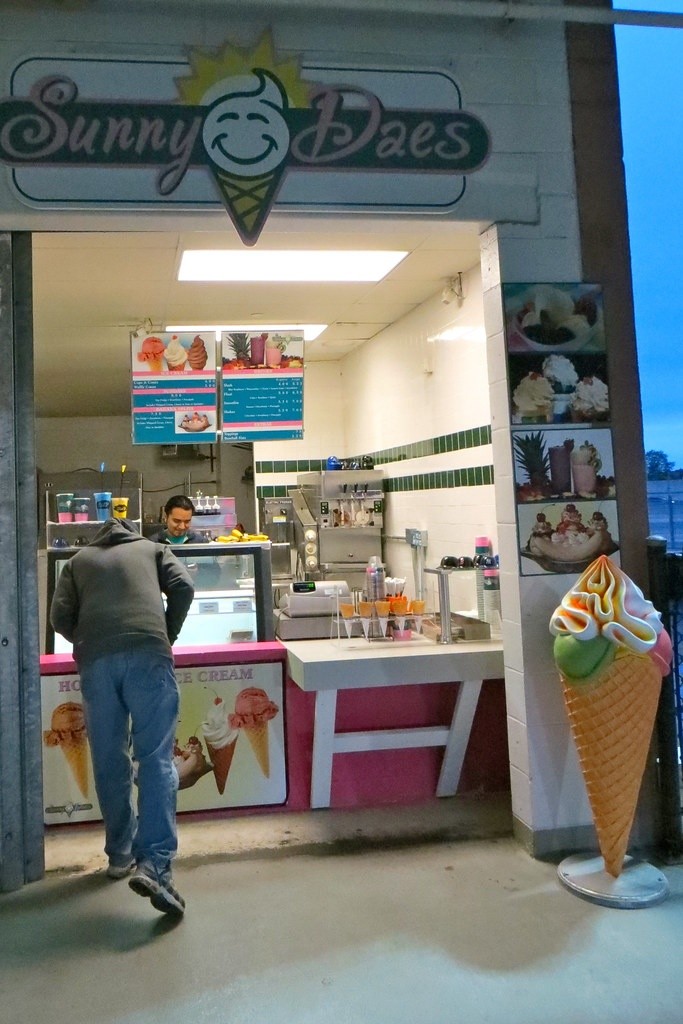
[{"x1": 46, "y1": 487, "x2": 143, "y2": 551}]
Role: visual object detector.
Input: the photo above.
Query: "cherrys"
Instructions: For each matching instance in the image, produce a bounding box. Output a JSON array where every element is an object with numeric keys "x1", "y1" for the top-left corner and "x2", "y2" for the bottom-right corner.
[
  {"x1": 174, "y1": 723, "x2": 206, "y2": 755},
  {"x1": 536, "y1": 503, "x2": 555, "y2": 522},
  {"x1": 593, "y1": 501, "x2": 603, "y2": 520},
  {"x1": 565, "y1": 502, "x2": 575, "y2": 513}
]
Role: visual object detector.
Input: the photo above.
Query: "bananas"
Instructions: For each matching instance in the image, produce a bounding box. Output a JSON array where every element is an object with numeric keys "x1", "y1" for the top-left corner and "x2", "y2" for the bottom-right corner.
[
  {"x1": 532, "y1": 533, "x2": 602, "y2": 560},
  {"x1": 176, "y1": 754, "x2": 198, "y2": 779},
  {"x1": 217, "y1": 529, "x2": 267, "y2": 543}
]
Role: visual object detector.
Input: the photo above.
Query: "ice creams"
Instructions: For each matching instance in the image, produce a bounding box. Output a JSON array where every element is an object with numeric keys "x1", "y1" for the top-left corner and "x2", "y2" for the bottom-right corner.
[
  {"x1": 137, "y1": 334, "x2": 208, "y2": 371},
  {"x1": 512, "y1": 354, "x2": 610, "y2": 424},
  {"x1": 44, "y1": 702, "x2": 93, "y2": 799},
  {"x1": 550, "y1": 554, "x2": 673, "y2": 878},
  {"x1": 201, "y1": 685, "x2": 280, "y2": 795}
]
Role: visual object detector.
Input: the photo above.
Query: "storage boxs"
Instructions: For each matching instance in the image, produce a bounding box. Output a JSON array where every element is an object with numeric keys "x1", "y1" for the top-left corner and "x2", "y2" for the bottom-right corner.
[{"x1": 409, "y1": 610, "x2": 490, "y2": 641}]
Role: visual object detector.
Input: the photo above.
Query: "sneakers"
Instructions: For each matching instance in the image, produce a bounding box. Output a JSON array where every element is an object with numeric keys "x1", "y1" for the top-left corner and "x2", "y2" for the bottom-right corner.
[
  {"x1": 129, "y1": 860, "x2": 186, "y2": 914},
  {"x1": 107, "y1": 854, "x2": 135, "y2": 878}
]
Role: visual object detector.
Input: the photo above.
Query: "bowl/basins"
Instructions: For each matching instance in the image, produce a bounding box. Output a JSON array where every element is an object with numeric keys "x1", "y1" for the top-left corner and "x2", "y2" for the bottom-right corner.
[
  {"x1": 52, "y1": 536, "x2": 90, "y2": 547},
  {"x1": 519, "y1": 540, "x2": 620, "y2": 572},
  {"x1": 510, "y1": 293, "x2": 603, "y2": 351}
]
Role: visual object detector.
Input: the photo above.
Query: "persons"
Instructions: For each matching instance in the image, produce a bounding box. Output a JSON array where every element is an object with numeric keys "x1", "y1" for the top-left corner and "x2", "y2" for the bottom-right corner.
[
  {"x1": 150, "y1": 494, "x2": 221, "y2": 587},
  {"x1": 50, "y1": 517, "x2": 194, "y2": 915}
]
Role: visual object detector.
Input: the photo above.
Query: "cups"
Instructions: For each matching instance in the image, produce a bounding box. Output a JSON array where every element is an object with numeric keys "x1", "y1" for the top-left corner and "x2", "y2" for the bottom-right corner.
[
  {"x1": 475, "y1": 553, "x2": 501, "y2": 641},
  {"x1": 55, "y1": 493, "x2": 90, "y2": 523},
  {"x1": 94, "y1": 492, "x2": 129, "y2": 521},
  {"x1": 365, "y1": 556, "x2": 389, "y2": 637},
  {"x1": 250, "y1": 337, "x2": 264, "y2": 364},
  {"x1": 548, "y1": 445, "x2": 571, "y2": 492},
  {"x1": 572, "y1": 459, "x2": 602, "y2": 495},
  {"x1": 266, "y1": 342, "x2": 286, "y2": 365}
]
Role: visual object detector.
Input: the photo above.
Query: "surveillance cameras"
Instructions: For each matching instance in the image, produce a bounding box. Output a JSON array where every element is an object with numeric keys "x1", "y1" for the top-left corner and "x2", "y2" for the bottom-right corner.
[{"x1": 442, "y1": 288, "x2": 457, "y2": 305}]
